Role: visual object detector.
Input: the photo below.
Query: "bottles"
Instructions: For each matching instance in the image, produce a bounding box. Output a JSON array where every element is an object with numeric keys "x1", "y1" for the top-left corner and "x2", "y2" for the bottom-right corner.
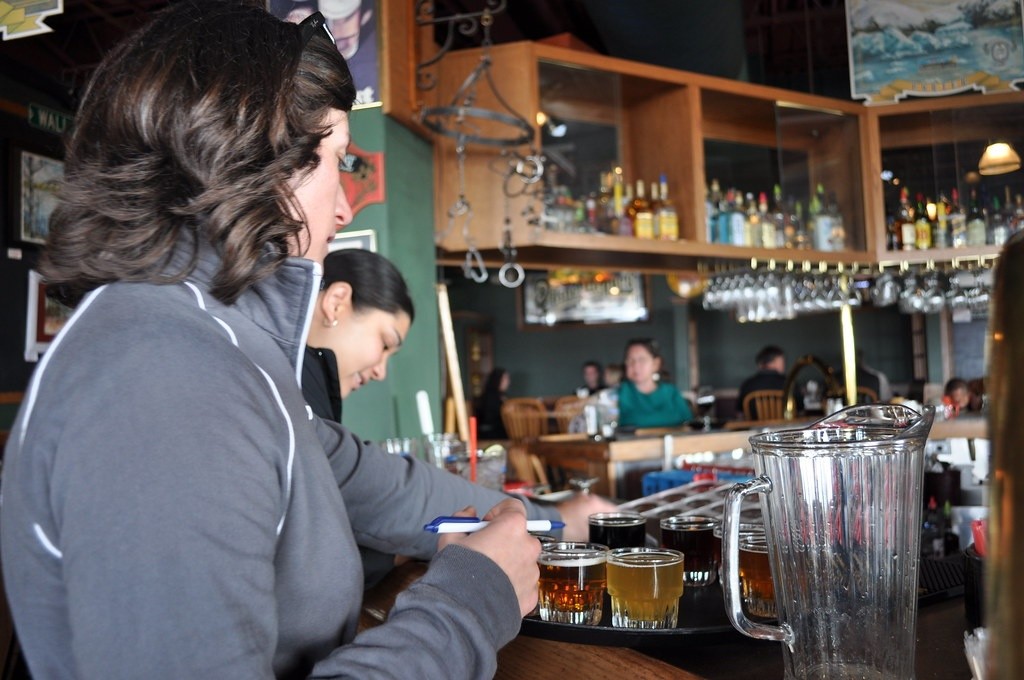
[
  {"x1": 705, "y1": 178, "x2": 845, "y2": 251},
  {"x1": 887, "y1": 180, "x2": 1023, "y2": 249},
  {"x1": 540, "y1": 166, "x2": 679, "y2": 241}
]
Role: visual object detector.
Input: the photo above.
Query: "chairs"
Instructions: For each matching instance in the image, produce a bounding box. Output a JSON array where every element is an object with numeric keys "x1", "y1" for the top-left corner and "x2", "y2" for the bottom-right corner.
[{"x1": 502, "y1": 387, "x2": 880, "y2": 496}]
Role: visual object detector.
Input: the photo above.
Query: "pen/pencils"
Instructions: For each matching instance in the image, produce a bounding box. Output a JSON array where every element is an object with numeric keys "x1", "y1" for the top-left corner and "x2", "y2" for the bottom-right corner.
[{"x1": 422, "y1": 514, "x2": 566, "y2": 534}]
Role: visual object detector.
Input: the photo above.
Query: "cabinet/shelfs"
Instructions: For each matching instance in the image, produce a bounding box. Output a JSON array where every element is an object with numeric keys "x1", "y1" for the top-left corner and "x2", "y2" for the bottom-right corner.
[{"x1": 435, "y1": 41, "x2": 1024, "y2": 276}]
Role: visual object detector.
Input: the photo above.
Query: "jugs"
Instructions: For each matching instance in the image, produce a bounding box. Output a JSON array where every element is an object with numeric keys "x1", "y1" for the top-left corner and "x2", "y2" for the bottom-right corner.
[{"x1": 719, "y1": 402, "x2": 937, "y2": 680}]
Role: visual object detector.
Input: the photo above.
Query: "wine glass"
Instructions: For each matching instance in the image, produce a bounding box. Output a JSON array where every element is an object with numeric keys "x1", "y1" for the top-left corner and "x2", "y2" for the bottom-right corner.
[{"x1": 694, "y1": 256, "x2": 997, "y2": 322}]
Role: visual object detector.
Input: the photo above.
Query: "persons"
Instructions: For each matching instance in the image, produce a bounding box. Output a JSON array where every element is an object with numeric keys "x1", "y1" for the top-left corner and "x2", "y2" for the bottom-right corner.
[
  {"x1": 0, "y1": 0, "x2": 623, "y2": 680},
  {"x1": 300, "y1": 248, "x2": 415, "y2": 593},
  {"x1": 473, "y1": 367, "x2": 514, "y2": 440},
  {"x1": 735, "y1": 345, "x2": 789, "y2": 422},
  {"x1": 944, "y1": 378, "x2": 972, "y2": 415},
  {"x1": 583, "y1": 338, "x2": 694, "y2": 434}
]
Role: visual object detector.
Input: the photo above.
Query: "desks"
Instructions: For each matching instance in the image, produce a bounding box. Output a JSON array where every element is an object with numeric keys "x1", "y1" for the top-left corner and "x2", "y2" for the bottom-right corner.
[{"x1": 536, "y1": 423, "x2": 755, "y2": 506}]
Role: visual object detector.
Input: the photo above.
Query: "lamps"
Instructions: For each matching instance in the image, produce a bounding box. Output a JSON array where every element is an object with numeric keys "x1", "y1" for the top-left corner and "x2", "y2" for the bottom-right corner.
[{"x1": 979, "y1": 143, "x2": 1021, "y2": 176}]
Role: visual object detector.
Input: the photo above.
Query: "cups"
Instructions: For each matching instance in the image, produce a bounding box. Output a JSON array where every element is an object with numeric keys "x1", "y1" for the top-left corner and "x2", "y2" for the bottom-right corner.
[
  {"x1": 527, "y1": 534, "x2": 559, "y2": 618},
  {"x1": 738, "y1": 533, "x2": 776, "y2": 618},
  {"x1": 661, "y1": 516, "x2": 724, "y2": 588},
  {"x1": 537, "y1": 541, "x2": 608, "y2": 624},
  {"x1": 608, "y1": 546, "x2": 681, "y2": 630},
  {"x1": 712, "y1": 523, "x2": 764, "y2": 589},
  {"x1": 590, "y1": 512, "x2": 645, "y2": 551},
  {"x1": 522, "y1": 268, "x2": 646, "y2": 325},
  {"x1": 382, "y1": 433, "x2": 465, "y2": 467}
]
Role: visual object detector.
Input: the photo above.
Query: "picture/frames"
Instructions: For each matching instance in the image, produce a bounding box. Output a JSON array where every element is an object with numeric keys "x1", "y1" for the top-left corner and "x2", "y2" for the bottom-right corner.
[
  {"x1": 327, "y1": 229, "x2": 376, "y2": 255},
  {"x1": 10, "y1": 145, "x2": 65, "y2": 247},
  {"x1": 22, "y1": 268, "x2": 77, "y2": 360}
]
